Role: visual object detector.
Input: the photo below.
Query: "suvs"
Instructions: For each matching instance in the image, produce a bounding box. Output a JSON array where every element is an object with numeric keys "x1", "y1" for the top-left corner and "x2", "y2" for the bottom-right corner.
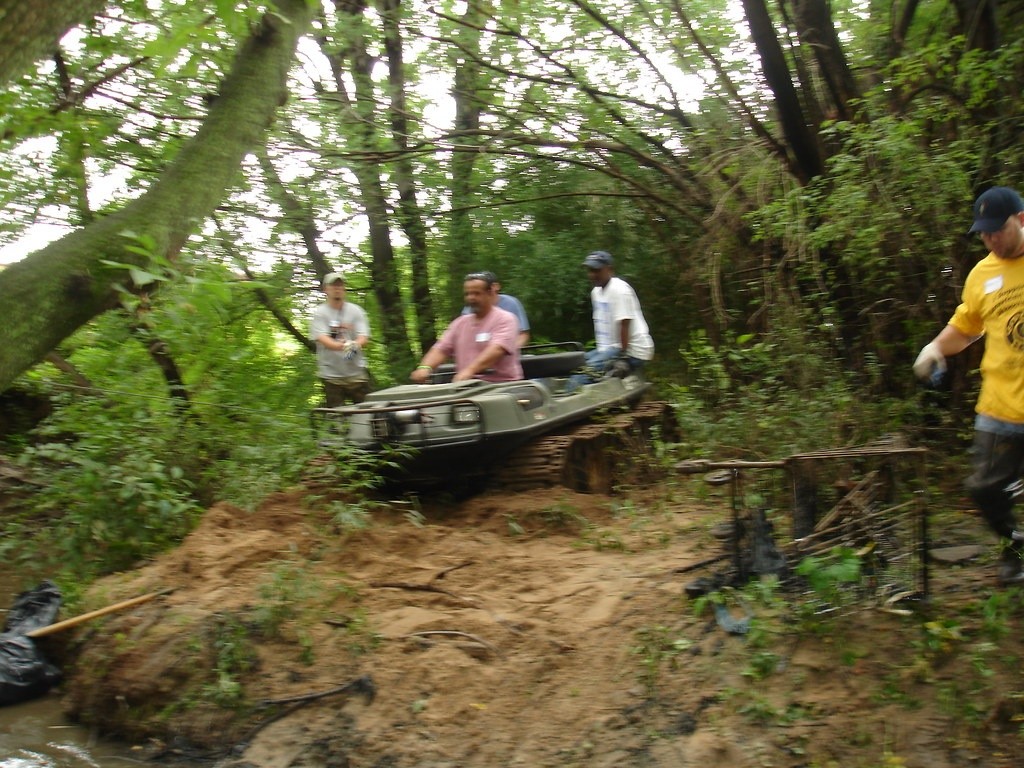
[{"x1": 307, "y1": 342, "x2": 684, "y2": 499}]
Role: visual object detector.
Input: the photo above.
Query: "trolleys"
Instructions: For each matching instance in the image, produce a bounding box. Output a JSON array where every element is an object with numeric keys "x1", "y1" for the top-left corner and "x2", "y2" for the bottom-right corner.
[{"x1": 675, "y1": 430, "x2": 930, "y2": 621}]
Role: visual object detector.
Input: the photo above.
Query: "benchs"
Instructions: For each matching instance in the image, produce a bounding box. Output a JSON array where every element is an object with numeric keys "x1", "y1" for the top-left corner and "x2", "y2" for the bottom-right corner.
[{"x1": 430, "y1": 352, "x2": 587, "y2": 382}]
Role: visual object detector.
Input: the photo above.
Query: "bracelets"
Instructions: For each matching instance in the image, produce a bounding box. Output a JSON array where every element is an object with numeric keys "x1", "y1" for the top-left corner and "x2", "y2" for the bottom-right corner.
[{"x1": 417, "y1": 366, "x2": 431, "y2": 369}]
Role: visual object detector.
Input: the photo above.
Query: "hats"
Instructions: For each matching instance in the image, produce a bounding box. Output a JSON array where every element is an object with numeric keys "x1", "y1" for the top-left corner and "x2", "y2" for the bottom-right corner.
[
  {"x1": 968, "y1": 186, "x2": 1024, "y2": 234},
  {"x1": 582, "y1": 250, "x2": 614, "y2": 269},
  {"x1": 473, "y1": 271, "x2": 497, "y2": 282},
  {"x1": 323, "y1": 273, "x2": 344, "y2": 284}
]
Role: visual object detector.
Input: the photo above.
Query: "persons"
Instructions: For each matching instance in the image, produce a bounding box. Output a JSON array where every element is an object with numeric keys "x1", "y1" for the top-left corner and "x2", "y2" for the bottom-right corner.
[
  {"x1": 309, "y1": 272, "x2": 372, "y2": 407},
  {"x1": 563, "y1": 251, "x2": 655, "y2": 391},
  {"x1": 461, "y1": 271, "x2": 530, "y2": 345},
  {"x1": 410, "y1": 273, "x2": 524, "y2": 383},
  {"x1": 913, "y1": 186, "x2": 1024, "y2": 581}
]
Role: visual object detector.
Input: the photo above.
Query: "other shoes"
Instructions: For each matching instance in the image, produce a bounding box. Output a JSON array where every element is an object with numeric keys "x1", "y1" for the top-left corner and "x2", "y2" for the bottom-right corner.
[{"x1": 997, "y1": 540, "x2": 1024, "y2": 583}]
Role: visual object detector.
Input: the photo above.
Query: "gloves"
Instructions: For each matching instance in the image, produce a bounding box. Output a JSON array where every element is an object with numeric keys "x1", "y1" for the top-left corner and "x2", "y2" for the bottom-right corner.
[
  {"x1": 912, "y1": 342, "x2": 947, "y2": 385},
  {"x1": 608, "y1": 355, "x2": 630, "y2": 379},
  {"x1": 343, "y1": 340, "x2": 362, "y2": 360}
]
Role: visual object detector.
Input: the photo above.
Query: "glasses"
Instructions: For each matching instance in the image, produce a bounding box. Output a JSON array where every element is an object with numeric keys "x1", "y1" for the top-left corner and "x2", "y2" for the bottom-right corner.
[
  {"x1": 976, "y1": 224, "x2": 1006, "y2": 240},
  {"x1": 468, "y1": 274, "x2": 485, "y2": 278}
]
